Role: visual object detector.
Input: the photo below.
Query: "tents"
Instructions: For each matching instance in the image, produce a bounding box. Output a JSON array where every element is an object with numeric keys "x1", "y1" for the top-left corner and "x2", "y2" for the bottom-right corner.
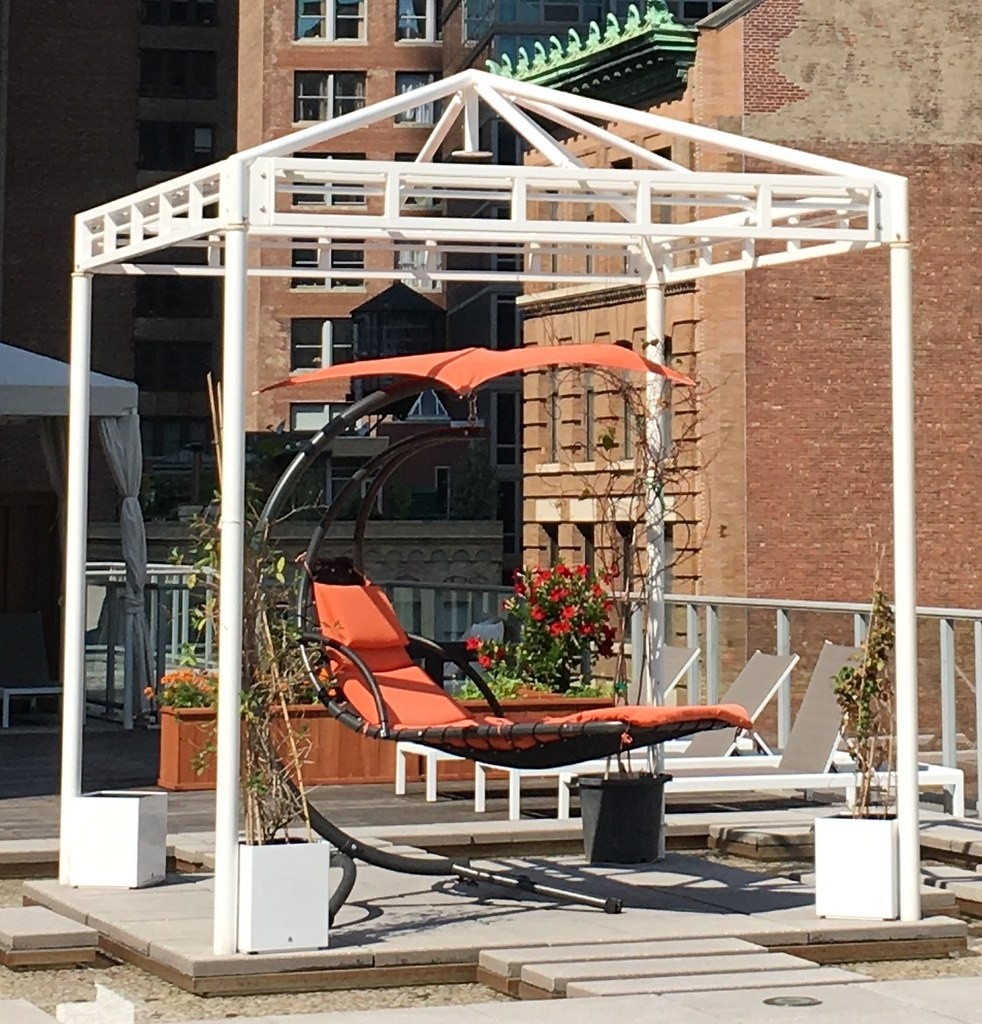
[{"x1": 0, "y1": 342, "x2": 154, "y2": 728}]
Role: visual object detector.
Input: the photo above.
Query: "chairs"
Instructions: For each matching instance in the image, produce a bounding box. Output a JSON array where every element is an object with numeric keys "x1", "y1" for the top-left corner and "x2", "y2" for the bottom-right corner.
[
  {"x1": 395, "y1": 642, "x2": 963, "y2": 820},
  {"x1": 240, "y1": 344, "x2": 753, "y2": 931},
  {"x1": 0, "y1": 610, "x2": 63, "y2": 729}
]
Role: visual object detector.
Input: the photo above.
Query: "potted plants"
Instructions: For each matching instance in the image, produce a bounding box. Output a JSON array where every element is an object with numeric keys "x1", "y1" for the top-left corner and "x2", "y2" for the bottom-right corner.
[
  {"x1": 528, "y1": 295, "x2": 722, "y2": 862},
  {"x1": 815, "y1": 540, "x2": 899, "y2": 920},
  {"x1": 167, "y1": 480, "x2": 328, "y2": 952}
]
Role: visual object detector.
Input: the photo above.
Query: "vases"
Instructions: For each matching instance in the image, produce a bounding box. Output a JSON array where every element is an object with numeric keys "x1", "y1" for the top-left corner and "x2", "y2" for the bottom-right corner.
[{"x1": 75, "y1": 790, "x2": 168, "y2": 890}]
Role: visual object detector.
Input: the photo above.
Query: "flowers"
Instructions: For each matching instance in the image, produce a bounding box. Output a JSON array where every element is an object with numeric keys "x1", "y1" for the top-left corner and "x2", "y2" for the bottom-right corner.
[{"x1": 144, "y1": 561, "x2": 620, "y2": 791}]
